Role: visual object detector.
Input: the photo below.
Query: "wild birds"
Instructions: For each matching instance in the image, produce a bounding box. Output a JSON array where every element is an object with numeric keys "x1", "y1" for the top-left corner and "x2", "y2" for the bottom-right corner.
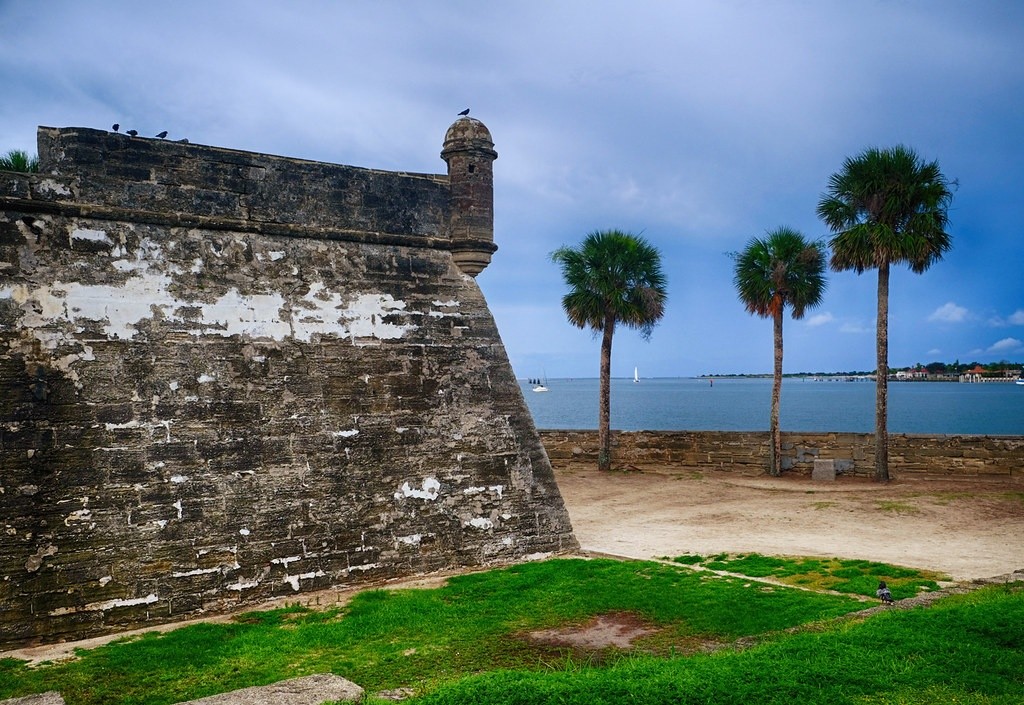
[
  {"x1": 457, "y1": 108, "x2": 469, "y2": 116},
  {"x1": 126, "y1": 130, "x2": 138, "y2": 136},
  {"x1": 112, "y1": 124, "x2": 120, "y2": 133},
  {"x1": 155, "y1": 131, "x2": 168, "y2": 139}
]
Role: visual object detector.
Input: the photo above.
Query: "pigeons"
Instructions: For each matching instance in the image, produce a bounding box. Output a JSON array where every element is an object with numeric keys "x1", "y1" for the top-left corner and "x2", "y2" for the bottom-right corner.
[{"x1": 877, "y1": 581, "x2": 897, "y2": 606}]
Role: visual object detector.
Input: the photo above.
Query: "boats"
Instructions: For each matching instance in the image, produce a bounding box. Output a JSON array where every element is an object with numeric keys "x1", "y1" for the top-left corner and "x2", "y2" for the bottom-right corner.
[
  {"x1": 1015, "y1": 379, "x2": 1024, "y2": 386},
  {"x1": 532, "y1": 386, "x2": 551, "y2": 392}
]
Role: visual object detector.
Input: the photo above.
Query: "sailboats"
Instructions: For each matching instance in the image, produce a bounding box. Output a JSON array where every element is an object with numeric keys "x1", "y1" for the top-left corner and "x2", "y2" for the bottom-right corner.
[{"x1": 633, "y1": 367, "x2": 640, "y2": 384}]
